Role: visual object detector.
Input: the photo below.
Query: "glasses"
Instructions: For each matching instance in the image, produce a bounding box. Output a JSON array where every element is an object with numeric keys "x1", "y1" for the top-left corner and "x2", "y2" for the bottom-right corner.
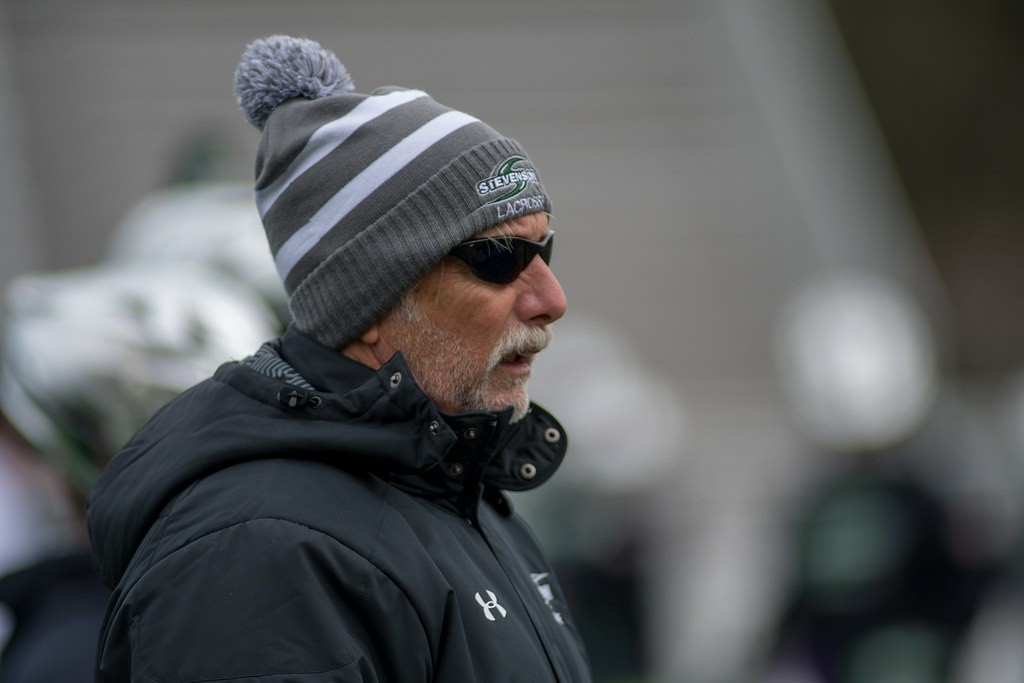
[{"x1": 449, "y1": 231, "x2": 555, "y2": 286}]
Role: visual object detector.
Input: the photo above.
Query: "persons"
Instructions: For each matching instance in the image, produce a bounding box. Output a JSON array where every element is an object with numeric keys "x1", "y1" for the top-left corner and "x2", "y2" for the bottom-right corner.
[{"x1": 83, "y1": 29, "x2": 595, "y2": 683}]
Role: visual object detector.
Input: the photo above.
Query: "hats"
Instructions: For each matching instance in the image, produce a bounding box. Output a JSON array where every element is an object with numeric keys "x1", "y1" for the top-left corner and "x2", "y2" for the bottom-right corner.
[{"x1": 233, "y1": 34, "x2": 552, "y2": 351}]
